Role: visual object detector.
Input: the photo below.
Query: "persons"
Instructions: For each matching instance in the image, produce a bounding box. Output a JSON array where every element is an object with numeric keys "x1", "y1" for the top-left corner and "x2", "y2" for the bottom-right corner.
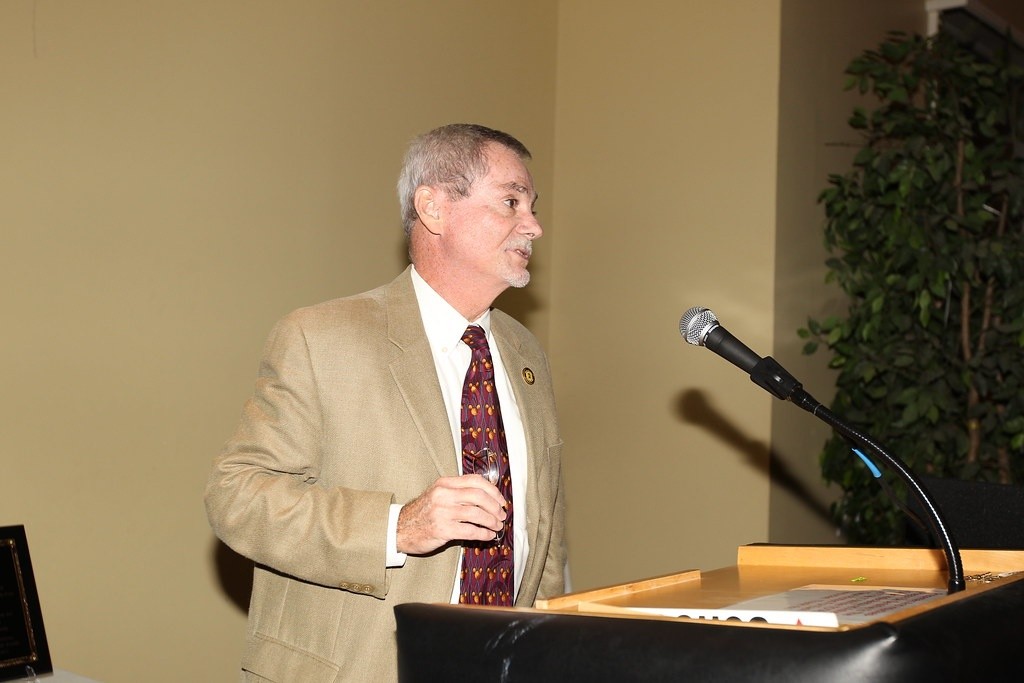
[{"x1": 205, "y1": 123, "x2": 569, "y2": 683}]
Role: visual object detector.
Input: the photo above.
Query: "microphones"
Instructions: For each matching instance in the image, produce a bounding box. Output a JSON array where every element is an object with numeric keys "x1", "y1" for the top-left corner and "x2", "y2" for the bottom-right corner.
[{"x1": 680, "y1": 306, "x2": 801, "y2": 400}]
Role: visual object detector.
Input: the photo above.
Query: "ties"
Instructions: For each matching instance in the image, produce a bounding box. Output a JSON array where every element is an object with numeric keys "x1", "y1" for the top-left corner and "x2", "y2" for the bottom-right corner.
[{"x1": 458, "y1": 325, "x2": 516, "y2": 607}]
